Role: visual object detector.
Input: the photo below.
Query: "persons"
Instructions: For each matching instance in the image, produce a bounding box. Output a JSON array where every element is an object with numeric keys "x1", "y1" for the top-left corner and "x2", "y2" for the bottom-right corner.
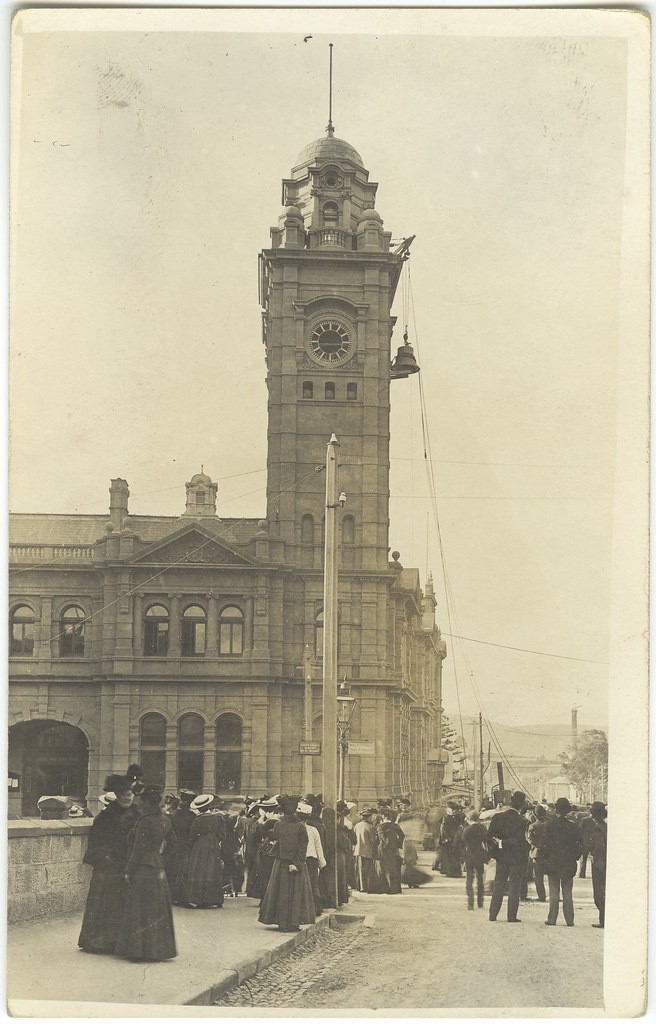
[
  {"x1": 487, "y1": 791, "x2": 532, "y2": 922},
  {"x1": 161, "y1": 788, "x2": 432, "y2": 931},
  {"x1": 585, "y1": 802, "x2": 607, "y2": 928},
  {"x1": 75, "y1": 764, "x2": 177, "y2": 961},
  {"x1": 535, "y1": 797, "x2": 582, "y2": 925},
  {"x1": 432, "y1": 799, "x2": 592, "y2": 910}
]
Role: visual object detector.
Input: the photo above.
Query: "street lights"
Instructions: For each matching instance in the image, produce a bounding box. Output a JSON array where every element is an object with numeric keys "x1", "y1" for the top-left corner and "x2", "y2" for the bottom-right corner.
[{"x1": 336, "y1": 672, "x2": 356, "y2": 825}]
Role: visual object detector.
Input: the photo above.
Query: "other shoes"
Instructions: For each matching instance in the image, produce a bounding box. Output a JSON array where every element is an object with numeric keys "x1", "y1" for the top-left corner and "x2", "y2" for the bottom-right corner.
[
  {"x1": 281, "y1": 924, "x2": 301, "y2": 933},
  {"x1": 407, "y1": 866, "x2": 604, "y2": 928}
]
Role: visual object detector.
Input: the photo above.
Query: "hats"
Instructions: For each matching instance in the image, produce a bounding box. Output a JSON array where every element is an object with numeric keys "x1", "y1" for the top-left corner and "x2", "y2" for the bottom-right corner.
[
  {"x1": 102, "y1": 775, "x2": 114, "y2": 791},
  {"x1": 296, "y1": 802, "x2": 312, "y2": 814},
  {"x1": 554, "y1": 797, "x2": 571, "y2": 815},
  {"x1": 511, "y1": 790, "x2": 526, "y2": 808},
  {"x1": 535, "y1": 803, "x2": 549, "y2": 816},
  {"x1": 276, "y1": 794, "x2": 302, "y2": 804},
  {"x1": 165, "y1": 792, "x2": 180, "y2": 811},
  {"x1": 111, "y1": 772, "x2": 137, "y2": 793},
  {"x1": 190, "y1": 794, "x2": 214, "y2": 809},
  {"x1": 99, "y1": 792, "x2": 116, "y2": 804},
  {"x1": 247, "y1": 802, "x2": 260, "y2": 814},
  {"x1": 589, "y1": 802, "x2": 607, "y2": 821},
  {"x1": 467, "y1": 810, "x2": 481, "y2": 820},
  {"x1": 68, "y1": 805, "x2": 83, "y2": 816},
  {"x1": 130, "y1": 781, "x2": 163, "y2": 795},
  {"x1": 362, "y1": 813, "x2": 371, "y2": 816},
  {"x1": 179, "y1": 789, "x2": 195, "y2": 802},
  {"x1": 257, "y1": 800, "x2": 278, "y2": 806}
]
